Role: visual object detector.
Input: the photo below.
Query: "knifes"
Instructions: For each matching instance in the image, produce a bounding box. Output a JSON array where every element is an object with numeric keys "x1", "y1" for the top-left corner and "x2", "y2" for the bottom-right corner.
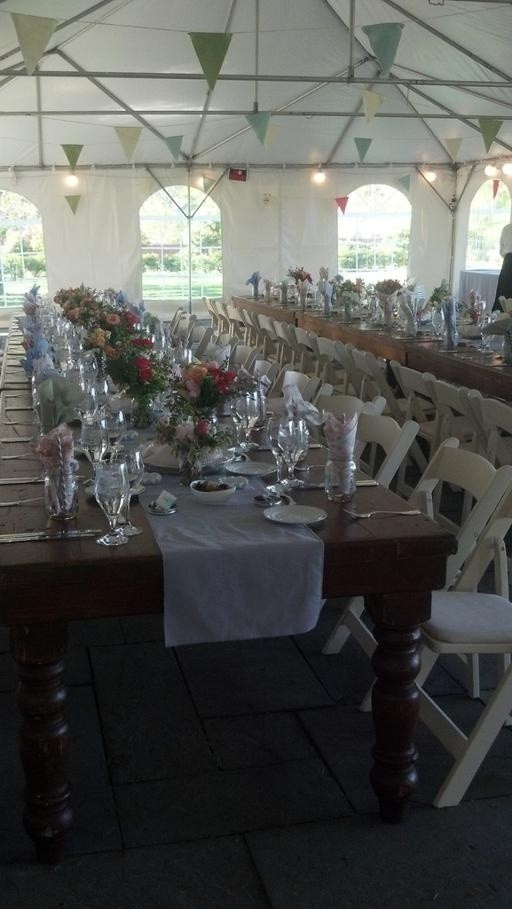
[{"x1": 0, "y1": 529, "x2": 101, "y2": 544}]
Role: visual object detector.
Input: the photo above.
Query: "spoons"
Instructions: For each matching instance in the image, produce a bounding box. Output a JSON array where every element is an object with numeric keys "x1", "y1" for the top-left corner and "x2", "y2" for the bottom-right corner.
[{"x1": 344, "y1": 507, "x2": 422, "y2": 519}]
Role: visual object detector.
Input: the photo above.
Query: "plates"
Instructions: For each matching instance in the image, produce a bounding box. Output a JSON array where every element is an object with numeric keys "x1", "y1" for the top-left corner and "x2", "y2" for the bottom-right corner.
[
  {"x1": 225, "y1": 461, "x2": 277, "y2": 477},
  {"x1": 263, "y1": 504, "x2": 329, "y2": 526}
]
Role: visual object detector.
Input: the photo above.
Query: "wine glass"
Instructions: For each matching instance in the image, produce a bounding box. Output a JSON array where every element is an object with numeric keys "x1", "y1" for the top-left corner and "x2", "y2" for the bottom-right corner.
[
  {"x1": 146, "y1": 312, "x2": 355, "y2": 501},
  {"x1": 23, "y1": 289, "x2": 145, "y2": 548}
]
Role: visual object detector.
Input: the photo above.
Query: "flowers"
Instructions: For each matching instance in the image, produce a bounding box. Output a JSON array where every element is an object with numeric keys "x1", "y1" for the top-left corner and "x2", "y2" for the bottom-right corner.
[{"x1": 285, "y1": 265, "x2": 486, "y2": 325}]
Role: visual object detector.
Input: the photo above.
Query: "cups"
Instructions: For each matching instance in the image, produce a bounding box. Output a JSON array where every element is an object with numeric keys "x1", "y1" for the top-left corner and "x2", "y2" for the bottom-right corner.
[{"x1": 247, "y1": 273, "x2": 512, "y2": 366}]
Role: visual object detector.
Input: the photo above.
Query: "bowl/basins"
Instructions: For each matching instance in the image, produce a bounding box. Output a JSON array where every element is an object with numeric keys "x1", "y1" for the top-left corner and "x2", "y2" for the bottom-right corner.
[{"x1": 190, "y1": 479, "x2": 237, "y2": 505}]
